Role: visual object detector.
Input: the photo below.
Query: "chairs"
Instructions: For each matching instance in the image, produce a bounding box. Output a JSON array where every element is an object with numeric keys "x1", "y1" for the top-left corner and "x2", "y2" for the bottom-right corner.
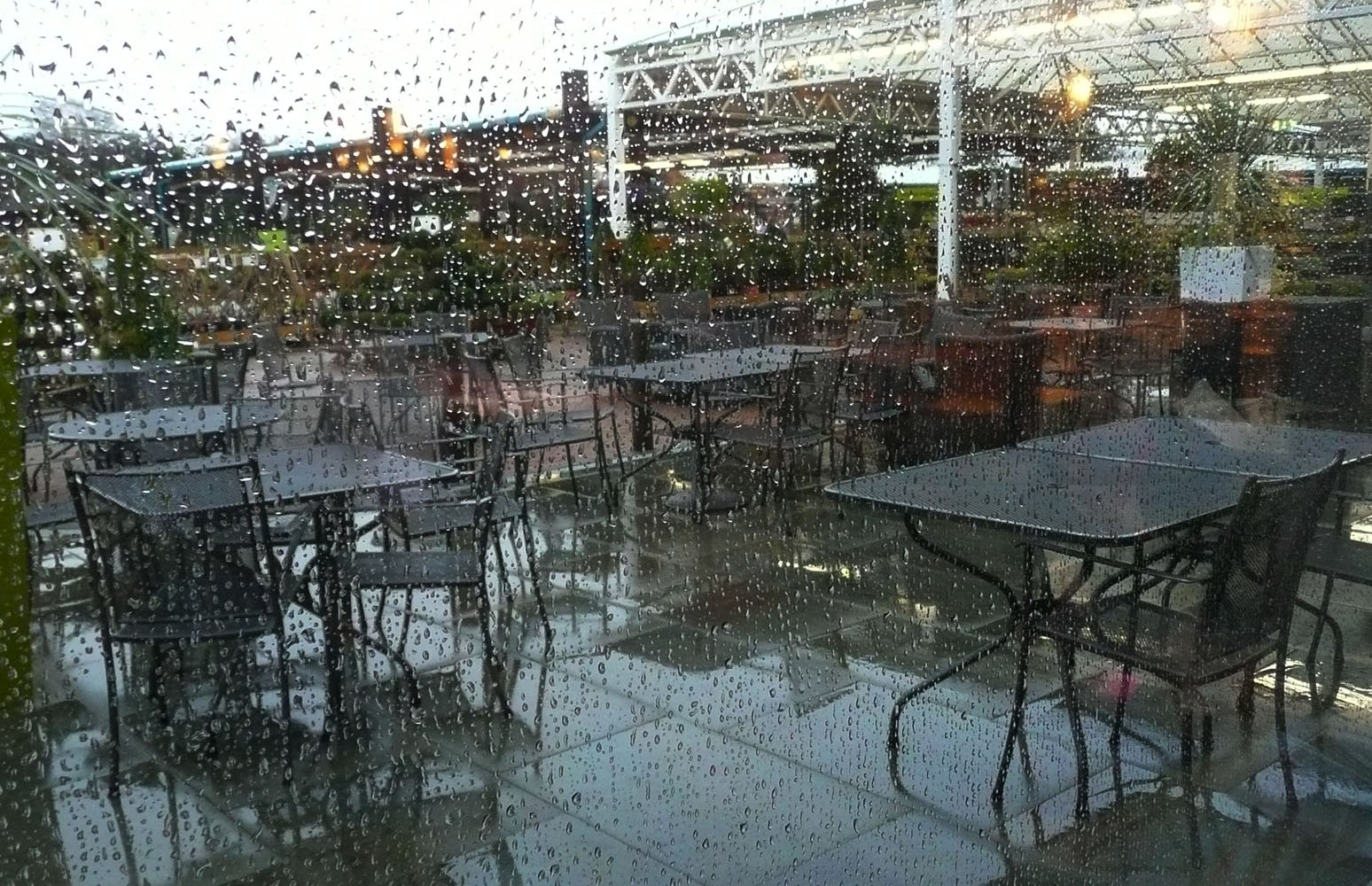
[{"x1": 25, "y1": 276, "x2": 1372, "y2": 825}]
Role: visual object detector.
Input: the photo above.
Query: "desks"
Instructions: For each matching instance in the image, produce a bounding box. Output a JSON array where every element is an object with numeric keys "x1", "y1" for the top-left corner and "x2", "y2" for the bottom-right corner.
[
  {"x1": 116, "y1": 441, "x2": 460, "y2": 722},
  {"x1": 686, "y1": 343, "x2": 883, "y2": 455},
  {"x1": 825, "y1": 447, "x2": 1282, "y2": 818},
  {"x1": 1018, "y1": 415, "x2": 1372, "y2": 718},
  {"x1": 585, "y1": 356, "x2": 808, "y2": 514},
  {"x1": 23, "y1": 360, "x2": 190, "y2": 491},
  {"x1": 360, "y1": 334, "x2": 436, "y2": 367},
  {"x1": 1272, "y1": 295, "x2": 1372, "y2": 389},
  {"x1": 47, "y1": 403, "x2": 284, "y2": 467},
  {"x1": 1011, "y1": 318, "x2": 1154, "y2": 423}
]
